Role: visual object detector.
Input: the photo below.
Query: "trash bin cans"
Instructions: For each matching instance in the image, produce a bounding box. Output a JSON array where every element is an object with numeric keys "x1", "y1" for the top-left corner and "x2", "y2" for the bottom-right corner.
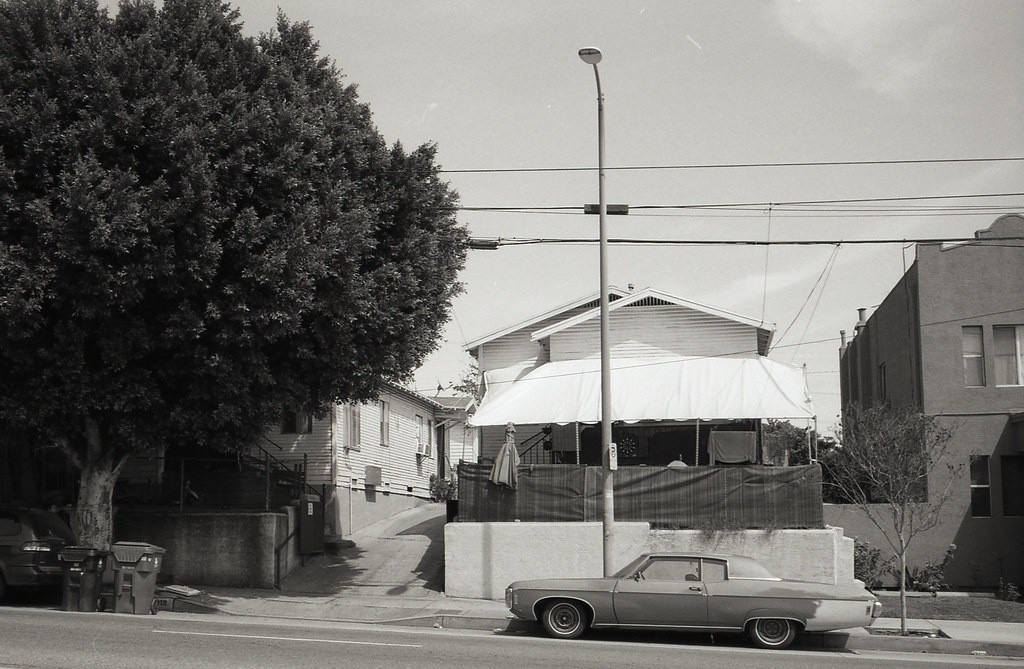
[
  {"x1": 56, "y1": 545, "x2": 113, "y2": 613},
  {"x1": 109, "y1": 540, "x2": 166, "y2": 615}
]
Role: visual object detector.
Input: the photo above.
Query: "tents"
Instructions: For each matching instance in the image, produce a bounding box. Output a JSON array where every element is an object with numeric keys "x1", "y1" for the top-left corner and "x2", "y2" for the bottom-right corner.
[{"x1": 465, "y1": 358, "x2": 817, "y2": 466}]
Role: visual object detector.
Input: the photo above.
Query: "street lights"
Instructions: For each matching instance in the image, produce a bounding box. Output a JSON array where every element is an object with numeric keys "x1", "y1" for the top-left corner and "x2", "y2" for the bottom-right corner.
[{"x1": 578, "y1": 45, "x2": 616, "y2": 580}]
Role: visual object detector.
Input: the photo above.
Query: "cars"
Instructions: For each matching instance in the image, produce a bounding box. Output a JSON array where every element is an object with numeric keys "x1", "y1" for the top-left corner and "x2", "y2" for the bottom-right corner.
[
  {"x1": 0, "y1": 500, "x2": 79, "y2": 607},
  {"x1": 505, "y1": 550, "x2": 885, "y2": 651}
]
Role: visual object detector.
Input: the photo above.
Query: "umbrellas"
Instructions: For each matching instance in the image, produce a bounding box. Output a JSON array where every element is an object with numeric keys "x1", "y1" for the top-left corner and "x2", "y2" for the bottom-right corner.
[{"x1": 489, "y1": 422, "x2": 521, "y2": 491}]
